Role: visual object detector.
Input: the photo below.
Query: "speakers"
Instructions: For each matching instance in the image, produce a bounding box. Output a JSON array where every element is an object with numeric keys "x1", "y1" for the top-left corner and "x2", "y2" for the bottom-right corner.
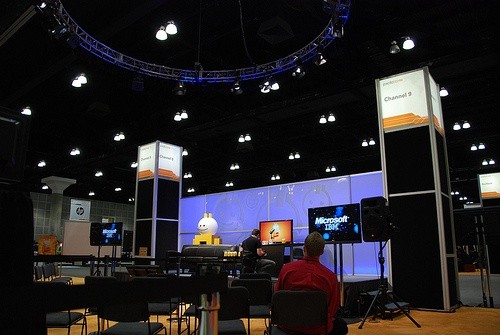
[{"x1": 361, "y1": 196, "x2": 391, "y2": 243}]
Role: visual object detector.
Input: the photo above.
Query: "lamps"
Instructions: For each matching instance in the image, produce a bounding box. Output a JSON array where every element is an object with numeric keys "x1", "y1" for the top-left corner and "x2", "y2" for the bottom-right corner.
[{"x1": 21, "y1": 20, "x2": 495, "y2": 201}]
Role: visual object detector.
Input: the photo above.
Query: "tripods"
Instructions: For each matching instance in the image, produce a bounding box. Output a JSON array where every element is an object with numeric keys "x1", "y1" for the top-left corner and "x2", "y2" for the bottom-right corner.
[{"x1": 358, "y1": 242, "x2": 421, "y2": 330}]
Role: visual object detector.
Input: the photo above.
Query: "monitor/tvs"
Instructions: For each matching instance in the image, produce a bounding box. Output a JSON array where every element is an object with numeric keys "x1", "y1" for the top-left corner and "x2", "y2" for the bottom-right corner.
[
  {"x1": 308, "y1": 203, "x2": 362, "y2": 244},
  {"x1": 90, "y1": 222, "x2": 123, "y2": 246},
  {"x1": 259, "y1": 219, "x2": 293, "y2": 245}
]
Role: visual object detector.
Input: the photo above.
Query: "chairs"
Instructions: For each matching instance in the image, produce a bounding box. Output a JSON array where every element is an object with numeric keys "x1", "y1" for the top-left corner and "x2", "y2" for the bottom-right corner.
[{"x1": 34, "y1": 261, "x2": 328, "y2": 335}]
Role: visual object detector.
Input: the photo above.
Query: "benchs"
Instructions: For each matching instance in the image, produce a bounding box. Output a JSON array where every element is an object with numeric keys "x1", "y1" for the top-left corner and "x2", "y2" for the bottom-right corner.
[{"x1": 179, "y1": 245, "x2": 239, "y2": 273}]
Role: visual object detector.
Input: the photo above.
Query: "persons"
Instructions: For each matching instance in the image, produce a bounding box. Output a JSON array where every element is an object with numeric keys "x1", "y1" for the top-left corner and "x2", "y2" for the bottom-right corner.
[
  {"x1": 273, "y1": 231, "x2": 348, "y2": 335},
  {"x1": 242, "y1": 229, "x2": 276, "y2": 273}
]
